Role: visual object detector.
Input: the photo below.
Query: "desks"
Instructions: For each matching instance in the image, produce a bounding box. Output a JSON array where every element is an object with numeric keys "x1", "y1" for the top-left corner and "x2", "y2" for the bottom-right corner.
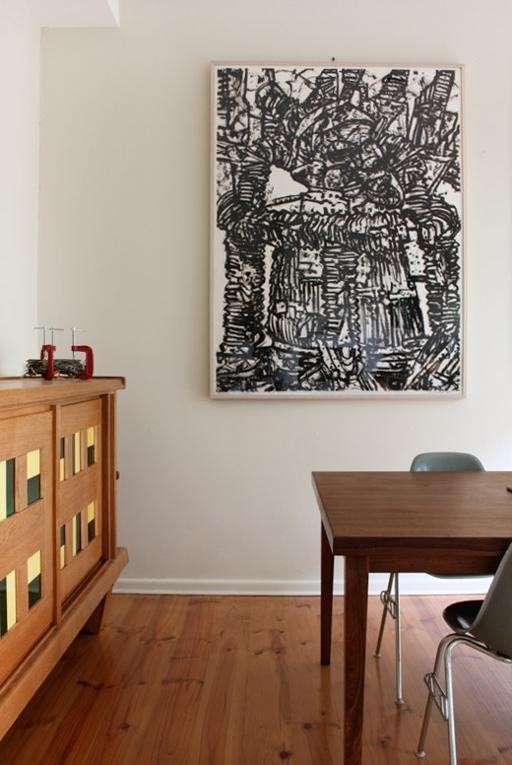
[{"x1": 312, "y1": 471, "x2": 511, "y2": 764}]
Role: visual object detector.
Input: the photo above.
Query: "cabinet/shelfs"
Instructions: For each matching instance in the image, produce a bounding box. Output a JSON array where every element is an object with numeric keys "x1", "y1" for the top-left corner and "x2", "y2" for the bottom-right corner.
[{"x1": 0, "y1": 376, "x2": 132, "y2": 742}]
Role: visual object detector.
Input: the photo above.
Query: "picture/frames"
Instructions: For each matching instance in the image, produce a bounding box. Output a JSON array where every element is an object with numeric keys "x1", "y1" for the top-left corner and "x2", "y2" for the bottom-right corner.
[{"x1": 208, "y1": 62, "x2": 467, "y2": 399}]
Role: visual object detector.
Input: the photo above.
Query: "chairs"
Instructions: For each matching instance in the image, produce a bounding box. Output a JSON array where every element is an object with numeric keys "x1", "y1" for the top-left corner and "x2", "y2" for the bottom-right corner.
[
  {"x1": 413, "y1": 543, "x2": 510, "y2": 763},
  {"x1": 372, "y1": 452, "x2": 485, "y2": 708}
]
